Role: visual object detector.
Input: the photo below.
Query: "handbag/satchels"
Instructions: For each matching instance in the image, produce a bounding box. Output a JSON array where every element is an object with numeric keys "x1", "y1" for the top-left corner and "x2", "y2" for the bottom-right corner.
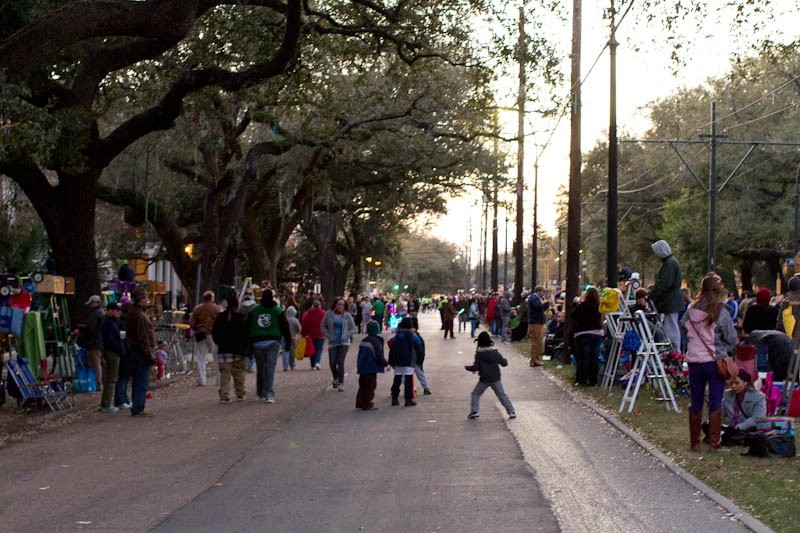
[
  {"x1": 715, "y1": 355, "x2": 739, "y2": 381},
  {"x1": 293, "y1": 334, "x2": 306, "y2": 360},
  {"x1": 194, "y1": 331, "x2": 207, "y2": 342},
  {"x1": 303, "y1": 336, "x2": 315, "y2": 357}
]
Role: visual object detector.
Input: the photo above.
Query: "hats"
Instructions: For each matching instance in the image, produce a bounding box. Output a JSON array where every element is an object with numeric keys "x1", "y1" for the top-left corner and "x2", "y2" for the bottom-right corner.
[
  {"x1": 365, "y1": 320, "x2": 380, "y2": 335},
  {"x1": 401, "y1": 318, "x2": 412, "y2": 329},
  {"x1": 411, "y1": 317, "x2": 418, "y2": 330},
  {"x1": 85, "y1": 295, "x2": 101, "y2": 304},
  {"x1": 131, "y1": 287, "x2": 151, "y2": 302},
  {"x1": 106, "y1": 303, "x2": 125, "y2": 311},
  {"x1": 474, "y1": 331, "x2": 494, "y2": 348},
  {"x1": 737, "y1": 366, "x2": 751, "y2": 386}
]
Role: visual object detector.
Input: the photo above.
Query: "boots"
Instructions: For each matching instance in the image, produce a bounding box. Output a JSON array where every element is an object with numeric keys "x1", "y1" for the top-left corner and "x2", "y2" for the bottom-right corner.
[
  {"x1": 708, "y1": 407, "x2": 726, "y2": 452},
  {"x1": 688, "y1": 406, "x2": 702, "y2": 451}
]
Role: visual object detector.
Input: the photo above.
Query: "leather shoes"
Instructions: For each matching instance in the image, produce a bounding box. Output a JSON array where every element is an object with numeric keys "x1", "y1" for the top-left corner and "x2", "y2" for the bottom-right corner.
[
  {"x1": 405, "y1": 399, "x2": 417, "y2": 406},
  {"x1": 391, "y1": 398, "x2": 400, "y2": 405},
  {"x1": 142, "y1": 407, "x2": 154, "y2": 412},
  {"x1": 134, "y1": 411, "x2": 154, "y2": 417}
]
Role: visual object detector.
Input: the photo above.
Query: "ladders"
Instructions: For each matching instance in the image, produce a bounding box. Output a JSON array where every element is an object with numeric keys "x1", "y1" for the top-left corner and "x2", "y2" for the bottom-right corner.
[
  {"x1": 600, "y1": 287, "x2": 659, "y2": 388},
  {"x1": 617, "y1": 309, "x2": 680, "y2": 414}
]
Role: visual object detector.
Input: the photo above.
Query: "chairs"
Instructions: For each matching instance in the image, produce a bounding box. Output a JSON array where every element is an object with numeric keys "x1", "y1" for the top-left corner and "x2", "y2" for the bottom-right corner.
[{"x1": 2, "y1": 351, "x2": 76, "y2": 416}]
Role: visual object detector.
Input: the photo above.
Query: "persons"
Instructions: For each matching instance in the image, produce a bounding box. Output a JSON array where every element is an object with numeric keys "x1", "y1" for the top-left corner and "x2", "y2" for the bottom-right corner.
[
  {"x1": 679, "y1": 273, "x2": 738, "y2": 452},
  {"x1": 239, "y1": 281, "x2": 528, "y2": 344},
  {"x1": 389, "y1": 317, "x2": 420, "y2": 406},
  {"x1": 321, "y1": 298, "x2": 355, "y2": 391},
  {"x1": 97, "y1": 302, "x2": 120, "y2": 412},
  {"x1": 190, "y1": 290, "x2": 221, "y2": 386},
  {"x1": 151, "y1": 340, "x2": 168, "y2": 382},
  {"x1": 282, "y1": 306, "x2": 302, "y2": 371},
  {"x1": 246, "y1": 288, "x2": 293, "y2": 403},
  {"x1": 73, "y1": 294, "x2": 104, "y2": 391},
  {"x1": 464, "y1": 331, "x2": 516, "y2": 419},
  {"x1": 355, "y1": 320, "x2": 388, "y2": 411},
  {"x1": 114, "y1": 302, "x2": 134, "y2": 408},
  {"x1": 527, "y1": 238, "x2": 800, "y2": 396},
  {"x1": 217, "y1": 295, "x2": 228, "y2": 309},
  {"x1": 211, "y1": 295, "x2": 248, "y2": 403},
  {"x1": 301, "y1": 299, "x2": 327, "y2": 370},
  {"x1": 700, "y1": 368, "x2": 766, "y2": 446},
  {"x1": 125, "y1": 287, "x2": 153, "y2": 417},
  {"x1": 408, "y1": 316, "x2": 432, "y2": 398}
]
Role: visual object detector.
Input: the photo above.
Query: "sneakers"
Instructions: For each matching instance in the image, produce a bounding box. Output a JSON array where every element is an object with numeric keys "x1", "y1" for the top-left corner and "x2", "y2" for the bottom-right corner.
[
  {"x1": 127, "y1": 401, "x2": 134, "y2": 408},
  {"x1": 101, "y1": 406, "x2": 119, "y2": 413},
  {"x1": 315, "y1": 362, "x2": 321, "y2": 370},
  {"x1": 260, "y1": 393, "x2": 275, "y2": 403},
  {"x1": 424, "y1": 389, "x2": 433, "y2": 395},
  {"x1": 467, "y1": 411, "x2": 480, "y2": 418},
  {"x1": 237, "y1": 395, "x2": 247, "y2": 400},
  {"x1": 312, "y1": 366, "x2": 317, "y2": 370},
  {"x1": 509, "y1": 412, "x2": 516, "y2": 418},
  {"x1": 116, "y1": 401, "x2": 130, "y2": 408},
  {"x1": 220, "y1": 399, "x2": 233, "y2": 403},
  {"x1": 198, "y1": 383, "x2": 207, "y2": 386}
]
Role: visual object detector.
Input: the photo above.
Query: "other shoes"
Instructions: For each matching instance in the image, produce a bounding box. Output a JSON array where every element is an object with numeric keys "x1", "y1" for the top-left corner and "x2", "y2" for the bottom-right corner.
[
  {"x1": 283, "y1": 368, "x2": 288, "y2": 371},
  {"x1": 369, "y1": 406, "x2": 379, "y2": 410},
  {"x1": 291, "y1": 365, "x2": 296, "y2": 370},
  {"x1": 333, "y1": 378, "x2": 345, "y2": 392}
]
